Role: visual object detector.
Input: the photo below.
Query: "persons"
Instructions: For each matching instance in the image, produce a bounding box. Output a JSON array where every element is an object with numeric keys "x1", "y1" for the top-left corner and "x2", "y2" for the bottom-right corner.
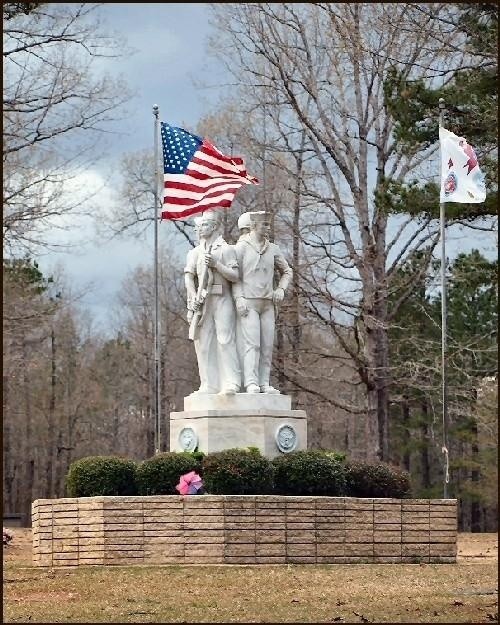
[{"x1": 184, "y1": 210, "x2": 293, "y2": 395}]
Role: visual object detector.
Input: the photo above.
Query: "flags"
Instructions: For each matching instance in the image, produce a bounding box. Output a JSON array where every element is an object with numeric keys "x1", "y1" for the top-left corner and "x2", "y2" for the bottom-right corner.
[
  {"x1": 158, "y1": 121, "x2": 260, "y2": 222},
  {"x1": 439, "y1": 127, "x2": 487, "y2": 204}
]
